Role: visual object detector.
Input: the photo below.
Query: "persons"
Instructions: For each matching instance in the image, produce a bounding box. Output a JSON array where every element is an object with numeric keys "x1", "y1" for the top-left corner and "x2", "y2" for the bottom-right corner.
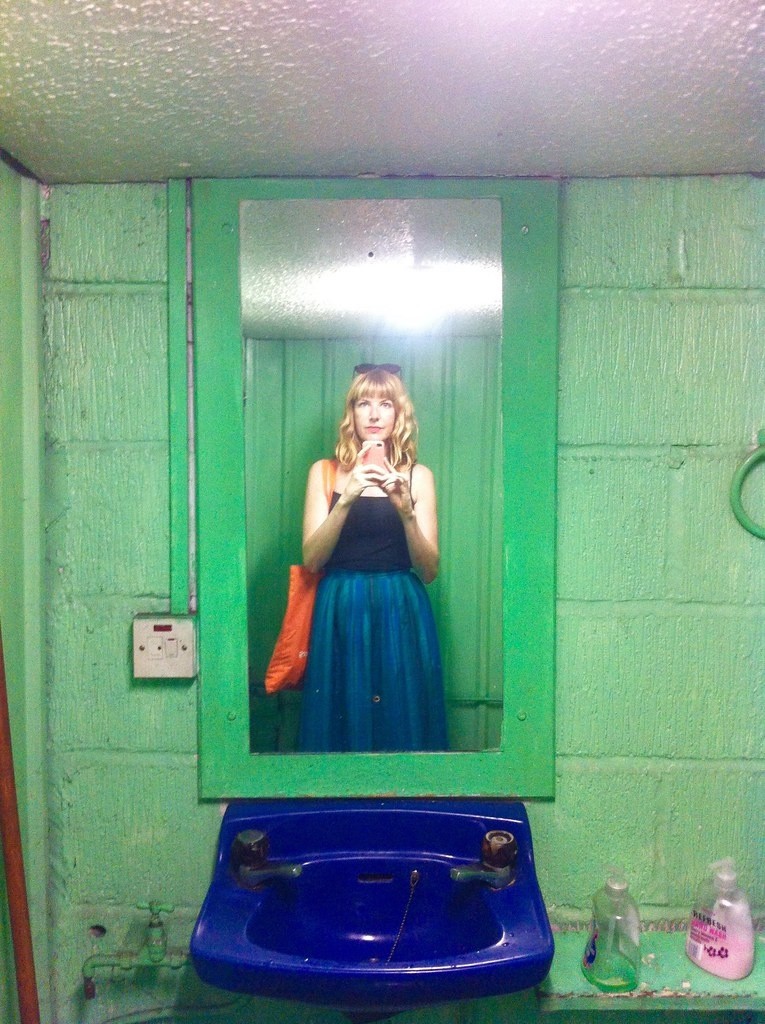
[{"x1": 302, "y1": 368, "x2": 441, "y2": 753}]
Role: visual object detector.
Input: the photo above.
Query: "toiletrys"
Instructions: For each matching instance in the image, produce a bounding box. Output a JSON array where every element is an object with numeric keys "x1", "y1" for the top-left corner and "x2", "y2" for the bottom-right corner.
[
  {"x1": 685, "y1": 858, "x2": 755, "y2": 981},
  {"x1": 578, "y1": 864, "x2": 641, "y2": 994}
]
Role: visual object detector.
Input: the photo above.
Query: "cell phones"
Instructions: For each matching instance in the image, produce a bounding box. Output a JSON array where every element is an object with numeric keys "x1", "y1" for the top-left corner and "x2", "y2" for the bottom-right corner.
[{"x1": 362, "y1": 440, "x2": 386, "y2": 483}]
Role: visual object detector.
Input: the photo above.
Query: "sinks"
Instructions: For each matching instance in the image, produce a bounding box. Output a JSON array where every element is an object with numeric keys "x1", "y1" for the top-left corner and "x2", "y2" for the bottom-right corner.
[{"x1": 190, "y1": 798, "x2": 554, "y2": 1012}]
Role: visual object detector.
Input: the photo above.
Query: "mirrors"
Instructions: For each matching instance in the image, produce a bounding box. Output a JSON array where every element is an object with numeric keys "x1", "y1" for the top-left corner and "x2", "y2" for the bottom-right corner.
[{"x1": 190, "y1": 177, "x2": 558, "y2": 799}]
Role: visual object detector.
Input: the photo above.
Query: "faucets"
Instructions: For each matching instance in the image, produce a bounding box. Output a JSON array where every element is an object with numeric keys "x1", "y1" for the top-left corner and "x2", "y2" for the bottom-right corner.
[
  {"x1": 449, "y1": 830, "x2": 520, "y2": 888},
  {"x1": 236, "y1": 829, "x2": 304, "y2": 890},
  {"x1": 82, "y1": 900, "x2": 182, "y2": 1000}
]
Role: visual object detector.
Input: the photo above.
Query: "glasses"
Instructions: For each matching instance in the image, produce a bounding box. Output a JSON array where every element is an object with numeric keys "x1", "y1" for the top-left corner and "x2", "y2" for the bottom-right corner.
[{"x1": 352, "y1": 364, "x2": 403, "y2": 383}]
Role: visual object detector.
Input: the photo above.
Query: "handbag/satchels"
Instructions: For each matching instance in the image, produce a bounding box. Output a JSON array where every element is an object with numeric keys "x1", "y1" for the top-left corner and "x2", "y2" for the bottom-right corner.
[{"x1": 264, "y1": 564, "x2": 324, "y2": 694}]
